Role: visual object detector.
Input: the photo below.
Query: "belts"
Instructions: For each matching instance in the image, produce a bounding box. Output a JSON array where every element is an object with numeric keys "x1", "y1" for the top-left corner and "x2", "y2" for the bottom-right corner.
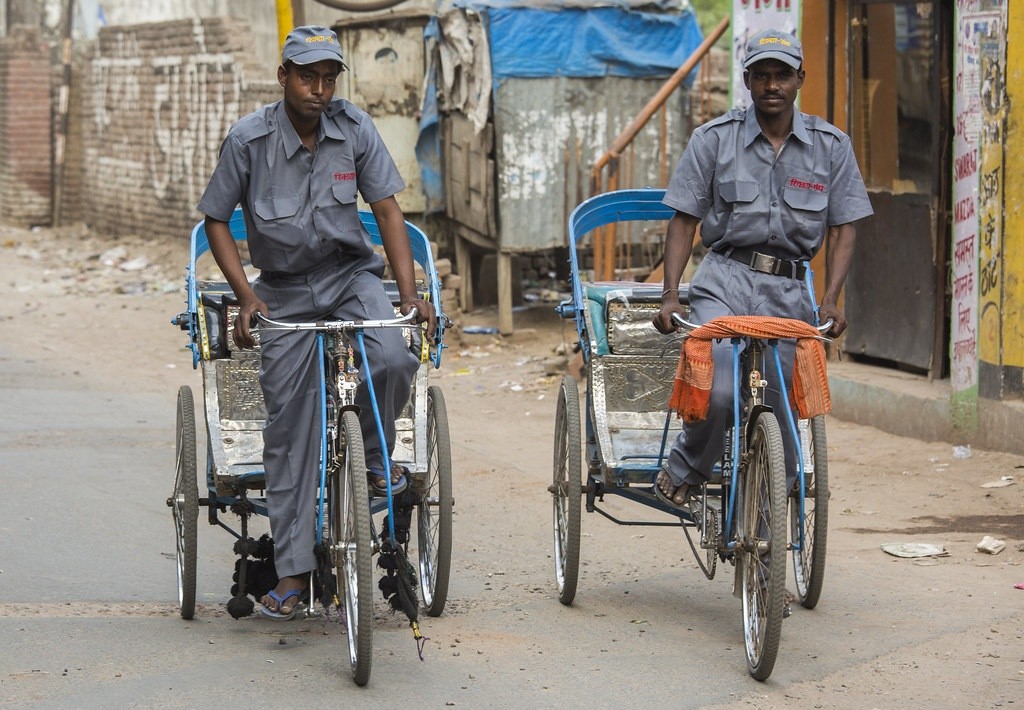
[{"x1": 719, "y1": 246, "x2": 806, "y2": 280}]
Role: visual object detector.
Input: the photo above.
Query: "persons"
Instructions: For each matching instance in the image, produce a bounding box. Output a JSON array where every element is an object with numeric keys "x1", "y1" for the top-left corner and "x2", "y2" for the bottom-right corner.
[
  {"x1": 653, "y1": 30, "x2": 873, "y2": 511},
  {"x1": 197, "y1": 26, "x2": 436, "y2": 623}
]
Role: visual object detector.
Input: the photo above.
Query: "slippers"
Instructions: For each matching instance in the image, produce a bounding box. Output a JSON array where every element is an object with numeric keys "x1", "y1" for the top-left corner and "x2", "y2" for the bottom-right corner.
[
  {"x1": 262, "y1": 588, "x2": 309, "y2": 621},
  {"x1": 367, "y1": 460, "x2": 407, "y2": 495}
]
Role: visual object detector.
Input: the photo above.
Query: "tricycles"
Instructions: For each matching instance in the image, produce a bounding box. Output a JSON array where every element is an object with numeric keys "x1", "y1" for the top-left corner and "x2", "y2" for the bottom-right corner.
[
  {"x1": 163, "y1": 199, "x2": 455, "y2": 686},
  {"x1": 547, "y1": 186, "x2": 833, "y2": 679}
]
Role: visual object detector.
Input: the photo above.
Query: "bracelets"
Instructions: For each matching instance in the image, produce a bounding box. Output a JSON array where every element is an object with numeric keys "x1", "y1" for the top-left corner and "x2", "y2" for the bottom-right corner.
[{"x1": 662, "y1": 289, "x2": 680, "y2": 298}]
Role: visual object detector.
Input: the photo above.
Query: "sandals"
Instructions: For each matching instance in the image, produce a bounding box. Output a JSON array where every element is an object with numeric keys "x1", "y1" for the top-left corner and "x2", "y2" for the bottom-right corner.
[{"x1": 656, "y1": 461, "x2": 692, "y2": 508}]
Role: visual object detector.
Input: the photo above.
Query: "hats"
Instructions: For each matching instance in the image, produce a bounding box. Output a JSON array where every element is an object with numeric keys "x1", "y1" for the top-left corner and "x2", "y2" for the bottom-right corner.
[
  {"x1": 741, "y1": 30, "x2": 804, "y2": 70},
  {"x1": 282, "y1": 26, "x2": 349, "y2": 68}
]
